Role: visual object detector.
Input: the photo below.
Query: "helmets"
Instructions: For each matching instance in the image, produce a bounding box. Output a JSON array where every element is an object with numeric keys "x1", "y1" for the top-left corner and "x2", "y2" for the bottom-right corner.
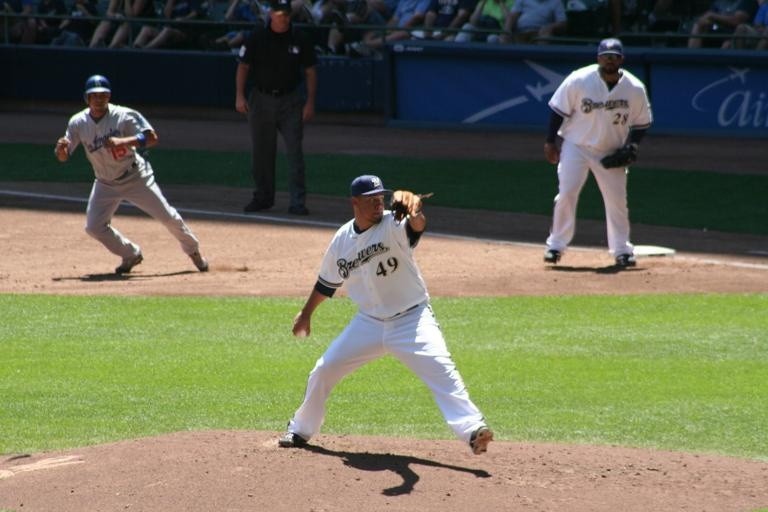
[
  {"x1": 597, "y1": 38, "x2": 624, "y2": 57},
  {"x1": 84, "y1": 73, "x2": 112, "y2": 95}
]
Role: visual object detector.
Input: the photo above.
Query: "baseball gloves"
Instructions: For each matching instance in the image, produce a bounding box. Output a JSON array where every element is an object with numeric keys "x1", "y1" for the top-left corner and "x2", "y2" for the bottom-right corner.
[
  {"x1": 601, "y1": 143, "x2": 637, "y2": 168},
  {"x1": 389, "y1": 190, "x2": 434, "y2": 226}
]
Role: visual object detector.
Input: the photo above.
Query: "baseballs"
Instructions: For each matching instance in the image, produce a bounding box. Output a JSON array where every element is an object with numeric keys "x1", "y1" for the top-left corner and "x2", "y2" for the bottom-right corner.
[{"x1": 296, "y1": 329, "x2": 307, "y2": 338}]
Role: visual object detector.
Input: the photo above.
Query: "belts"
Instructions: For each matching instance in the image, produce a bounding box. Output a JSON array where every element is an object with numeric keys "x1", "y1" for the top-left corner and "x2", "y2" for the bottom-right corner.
[{"x1": 259, "y1": 86, "x2": 296, "y2": 97}]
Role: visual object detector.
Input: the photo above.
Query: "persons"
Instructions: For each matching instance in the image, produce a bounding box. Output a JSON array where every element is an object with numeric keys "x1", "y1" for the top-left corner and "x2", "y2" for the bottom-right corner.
[
  {"x1": 277, "y1": 173, "x2": 492, "y2": 456},
  {"x1": 234, "y1": 0, "x2": 318, "y2": 215},
  {"x1": 54, "y1": 74, "x2": 209, "y2": 273},
  {"x1": 286, "y1": 1, "x2": 595, "y2": 58},
  {"x1": 542, "y1": 37, "x2": 655, "y2": 268},
  {"x1": 3, "y1": 2, "x2": 271, "y2": 51},
  {"x1": 611, "y1": 1, "x2": 768, "y2": 49}
]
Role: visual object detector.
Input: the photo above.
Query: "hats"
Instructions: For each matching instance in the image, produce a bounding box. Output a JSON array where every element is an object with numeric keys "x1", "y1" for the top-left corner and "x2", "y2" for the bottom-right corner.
[{"x1": 350, "y1": 174, "x2": 394, "y2": 197}]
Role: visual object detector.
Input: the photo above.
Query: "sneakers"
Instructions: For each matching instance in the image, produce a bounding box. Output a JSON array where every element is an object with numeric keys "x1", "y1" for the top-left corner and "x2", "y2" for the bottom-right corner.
[
  {"x1": 115, "y1": 249, "x2": 144, "y2": 274},
  {"x1": 190, "y1": 249, "x2": 208, "y2": 271},
  {"x1": 469, "y1": 425, "x2": 494, "y2": 456},
  {"x1": 543, "y1": 247, "x2": 563, "y2": 266},
  {"x1": 289, "y1": 204, "x2": 308, "y2": 215},
  {"x1": 616, "y1": 253, "x2": 636, "y2": 267},
  {"x1": 244, "y1": 200, "x2": 275, "y2": 211},
  {"x1": 279, "y1": 431, "x2": 306, "y2": 448}
]
